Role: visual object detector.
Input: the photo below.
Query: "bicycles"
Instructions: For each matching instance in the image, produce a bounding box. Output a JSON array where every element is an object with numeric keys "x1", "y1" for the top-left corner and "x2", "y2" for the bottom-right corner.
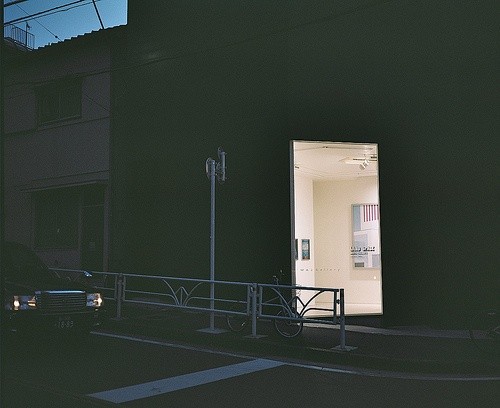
[{"x1": 225, "y1": 270, "x2": 304, "y2": 337}]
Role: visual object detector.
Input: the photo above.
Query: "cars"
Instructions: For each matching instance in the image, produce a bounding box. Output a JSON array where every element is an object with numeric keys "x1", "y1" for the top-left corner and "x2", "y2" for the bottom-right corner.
[{"x1": 2, "y1": 242, "x2": 106, "y2": 334}]
